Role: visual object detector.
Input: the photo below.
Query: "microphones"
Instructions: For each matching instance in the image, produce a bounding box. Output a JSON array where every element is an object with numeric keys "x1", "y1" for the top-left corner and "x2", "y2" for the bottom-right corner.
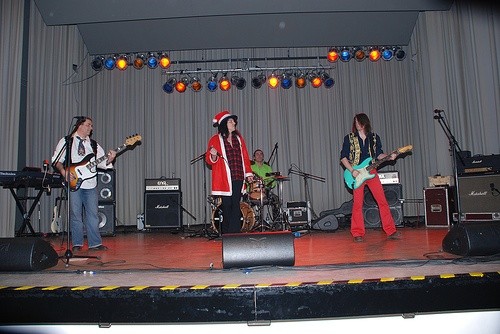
[
  {"x1": 434, "y1": 109, "x2": 444, "y2": 113},
  {"x1": 74, "y1": 116, "x2": 86, "y2": 122},
  {"x1": 490, "y1": 183, "x2": 494, "y2": 194},
  {"x1": 209, "y1": 147, "x2": 224, "y2": 159},
  {"x1": 275, "y1": 142, "x2": 278, "y2": 153},
  {"x1": 288, "y1": 167, "x2": 292, "y2": 175}
]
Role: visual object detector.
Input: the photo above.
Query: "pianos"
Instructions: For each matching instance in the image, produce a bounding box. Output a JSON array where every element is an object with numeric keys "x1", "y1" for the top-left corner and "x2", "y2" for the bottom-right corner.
[{"x1": 0, "y1": 166, "x2": 67, "y2": 239}]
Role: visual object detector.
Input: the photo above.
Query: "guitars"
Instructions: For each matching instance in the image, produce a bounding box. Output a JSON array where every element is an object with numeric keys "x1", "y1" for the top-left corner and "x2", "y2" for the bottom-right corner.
[
  {"x1": 50, "y1": 188, "x2": 64, "y2": 233},
  {"x1": 342, "y1": 143, "x2": 413, "y2": 190},
  {"x1": 59, "y1": 132, "x2": 142, "y2": 192}
]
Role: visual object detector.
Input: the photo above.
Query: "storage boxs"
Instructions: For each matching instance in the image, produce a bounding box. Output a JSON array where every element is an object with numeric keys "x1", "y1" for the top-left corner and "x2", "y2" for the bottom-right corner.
[{"x1": 423, "y1": 186, "x2": 455, "y2": 228}]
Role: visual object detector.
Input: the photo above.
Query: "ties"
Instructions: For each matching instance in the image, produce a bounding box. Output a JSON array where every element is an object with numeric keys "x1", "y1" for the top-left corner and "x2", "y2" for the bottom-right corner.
[{"x1": 76, "y1": 136, "x2": 86, "y2": 156}]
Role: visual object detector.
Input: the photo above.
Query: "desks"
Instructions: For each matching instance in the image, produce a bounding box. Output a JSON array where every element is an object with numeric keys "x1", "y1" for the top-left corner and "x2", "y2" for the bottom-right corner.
[{"x1": 0, "y1": 177, "x2": 62, "y2": 235}]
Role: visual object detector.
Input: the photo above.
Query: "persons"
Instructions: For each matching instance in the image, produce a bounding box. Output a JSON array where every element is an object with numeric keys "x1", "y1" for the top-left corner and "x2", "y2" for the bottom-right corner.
[
  {"x1": 243, "y1": 149, "x2": 285, "y2": 231},
  {"x1": 52, "y1": 117, "x2": 116, "y2": 252},
  {"x1": 340, "y1": 113, "x2": 402, "y2": 241},
  {"x1": 205, "y1": 111, "x2": 254, "y2": 234}
]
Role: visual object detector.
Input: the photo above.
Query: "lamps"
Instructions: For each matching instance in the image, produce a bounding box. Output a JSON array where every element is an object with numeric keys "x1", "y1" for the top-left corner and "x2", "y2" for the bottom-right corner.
[{"x1": 91, "y1": 46, "x2": 407, "y2": 94}]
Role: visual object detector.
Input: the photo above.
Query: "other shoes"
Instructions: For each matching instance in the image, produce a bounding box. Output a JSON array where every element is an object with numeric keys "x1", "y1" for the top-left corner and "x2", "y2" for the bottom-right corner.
[
  {"x1": 355, "y1": 236, "x2": 362, "y2": 241},
  {"x1": 388, "y1": 232, "x2": 402, "y2": 240},
  {"x1": 72, "y1": 246, "x2": 80, "y2": 251},
  {"x1": 89, "y1": 245, "x2": 108, "y2": 251}
]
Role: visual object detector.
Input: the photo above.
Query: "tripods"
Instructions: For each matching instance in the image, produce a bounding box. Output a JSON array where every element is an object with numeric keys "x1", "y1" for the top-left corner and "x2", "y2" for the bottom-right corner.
[
  {"x1": 57, "y1": 119, "x2": 100, "y2": 268},
  {"x1": 250, "y1": 190, "x2": 276, "y2": 232}
]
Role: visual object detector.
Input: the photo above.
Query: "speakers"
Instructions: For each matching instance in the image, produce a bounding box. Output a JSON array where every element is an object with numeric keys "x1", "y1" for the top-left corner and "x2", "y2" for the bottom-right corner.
[
  {"x1": 144, "y1": 192, "x2": 183, "y2": 230},
  {"x1": 0, "y1": 236, "x2": 59, "y2": 272},
  {"x1": 221, "y1": 232, "x2": 296, "y2": 270},
  {"x1": 363, "y1": 184, "x2": 403, "y2": 229},
  {"x1": 442, "y1": 220, "x2": 500, "y2": 257},
  {"x1": 311, "y1": 213, "x2": 340, "y2": 231},
  {"x1": 457, "y1": 174, "x2": 500, "y2": 214},
  {"x1": 97, "y1": 168, "x2": 116, "y2": 204},
  {"x1": 82, "y1": 203, "x2": 115, "y2": 238}
]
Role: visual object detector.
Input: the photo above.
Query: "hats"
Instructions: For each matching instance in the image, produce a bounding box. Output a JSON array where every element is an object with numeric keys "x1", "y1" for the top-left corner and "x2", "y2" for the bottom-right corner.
[{"x1": 213, "y1": 111, "x2": 237, "y2": 126}]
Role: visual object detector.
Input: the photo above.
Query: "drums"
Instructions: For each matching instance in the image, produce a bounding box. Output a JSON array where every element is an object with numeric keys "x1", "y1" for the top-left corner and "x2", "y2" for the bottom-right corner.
[
  {"x1": 211, "y1": 201, "x2": 256, "y2": 235},
  {"x1": 249, "y1": 179, "x2": 267, "y2": 200}
]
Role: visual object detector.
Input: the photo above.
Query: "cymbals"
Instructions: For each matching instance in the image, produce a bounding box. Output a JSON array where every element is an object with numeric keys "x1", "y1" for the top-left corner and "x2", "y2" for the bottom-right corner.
[{"x1": 267, "y1": 175, "x2": 292, "y2": 180}]
